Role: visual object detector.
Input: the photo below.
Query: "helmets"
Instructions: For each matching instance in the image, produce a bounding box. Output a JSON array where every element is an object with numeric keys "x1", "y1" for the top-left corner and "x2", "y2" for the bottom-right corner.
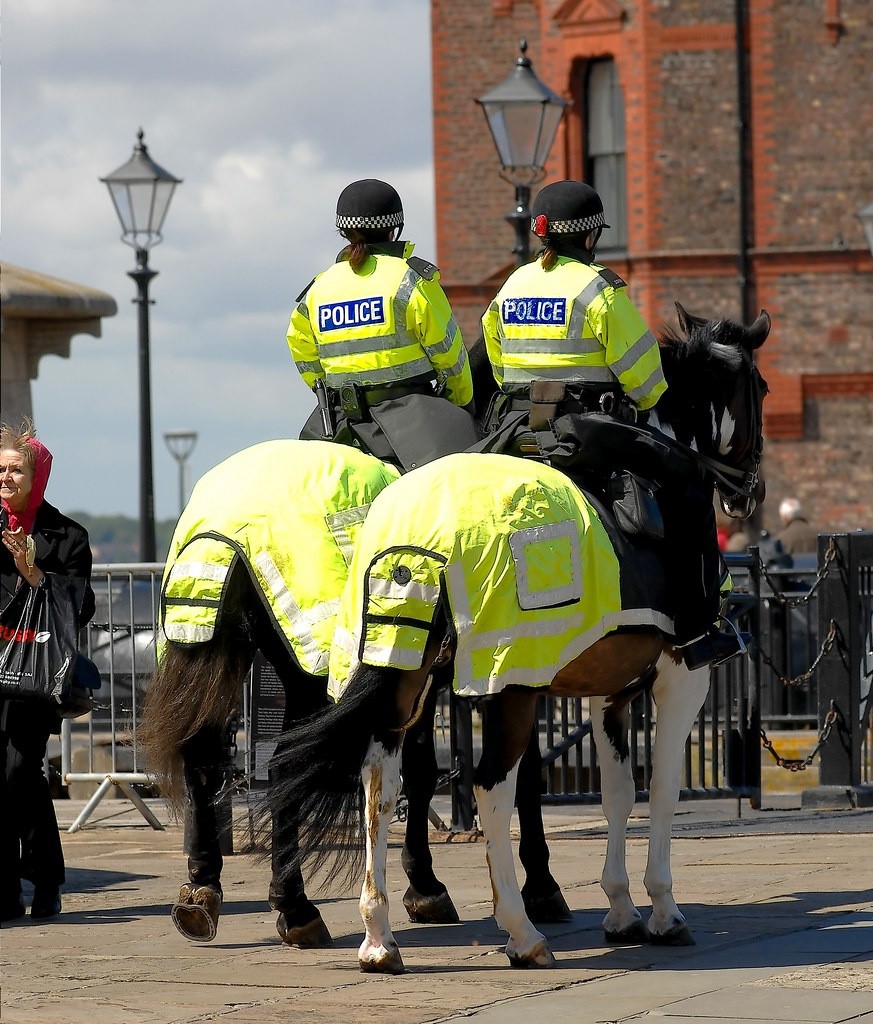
[
  {"x1": 530, "y1": 181, "x2": 613, "y2": 238},
  {"x1": 337, "y1": 179, "x2": 404, "y2": 233}
]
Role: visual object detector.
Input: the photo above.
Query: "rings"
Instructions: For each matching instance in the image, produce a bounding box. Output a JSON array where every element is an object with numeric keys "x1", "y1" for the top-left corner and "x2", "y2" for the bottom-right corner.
[
  {"x1": 15, "y1": 545, "x2": 20, "y2": 549},
  {"x1": 20, "y1": 540, "x2": 24, "y2": 546}
]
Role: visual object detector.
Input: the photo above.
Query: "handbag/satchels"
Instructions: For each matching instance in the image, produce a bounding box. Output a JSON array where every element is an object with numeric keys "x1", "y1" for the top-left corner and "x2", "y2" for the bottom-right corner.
[{"x1": 1, "y1": 571, "x2": 88, "y2": 698}]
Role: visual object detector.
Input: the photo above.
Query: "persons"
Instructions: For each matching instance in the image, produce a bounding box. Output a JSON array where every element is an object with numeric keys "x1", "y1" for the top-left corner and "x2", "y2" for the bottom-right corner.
[
  {"x1": 480, "y1": 181, "x2": 753, "y2": 670},
  {"x1": 774, "y1": 498, "x2": 820, "y2": 553},
  {"x1": 0, "y1": 416, "x2": 95, "y2": 922},
  {"x1": 287, "y1": 180, "x2": 479, "y2": 473}
]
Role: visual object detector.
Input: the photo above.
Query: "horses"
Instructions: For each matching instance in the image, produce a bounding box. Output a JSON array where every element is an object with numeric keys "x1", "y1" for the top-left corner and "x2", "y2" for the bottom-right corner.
[{"x1": 121, "y1": 298, "x2": 770, "y2": 975}]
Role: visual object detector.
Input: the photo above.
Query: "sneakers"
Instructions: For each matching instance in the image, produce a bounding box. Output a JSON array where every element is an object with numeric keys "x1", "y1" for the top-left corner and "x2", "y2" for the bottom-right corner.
[
  {"x1": 682, "y1": 628, "x2": 755, "y2": 670},
  {"x1": 0, "y1": 895, "x2": 26, "y2": 921},
  {"x1": 30, "y1": 890, "x2": 61, "y2": 919}
]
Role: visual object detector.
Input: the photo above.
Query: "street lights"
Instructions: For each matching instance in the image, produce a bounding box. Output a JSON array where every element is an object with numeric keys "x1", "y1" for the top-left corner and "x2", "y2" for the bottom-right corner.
[
  {"x1": 98, "y1": 124, "x2": 182, "y2": 565},
  {"x1": 474, "y1": 39, "x2": 570, "y2": 423},
  {"x1": 163, "y1": 427, "x2": 196, "y2": 529}
]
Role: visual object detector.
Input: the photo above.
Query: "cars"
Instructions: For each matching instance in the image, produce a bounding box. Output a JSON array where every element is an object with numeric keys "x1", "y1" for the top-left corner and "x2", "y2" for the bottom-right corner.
[{"x1": 91, "y1": 578, "x2": 358, "y2": 789}]
